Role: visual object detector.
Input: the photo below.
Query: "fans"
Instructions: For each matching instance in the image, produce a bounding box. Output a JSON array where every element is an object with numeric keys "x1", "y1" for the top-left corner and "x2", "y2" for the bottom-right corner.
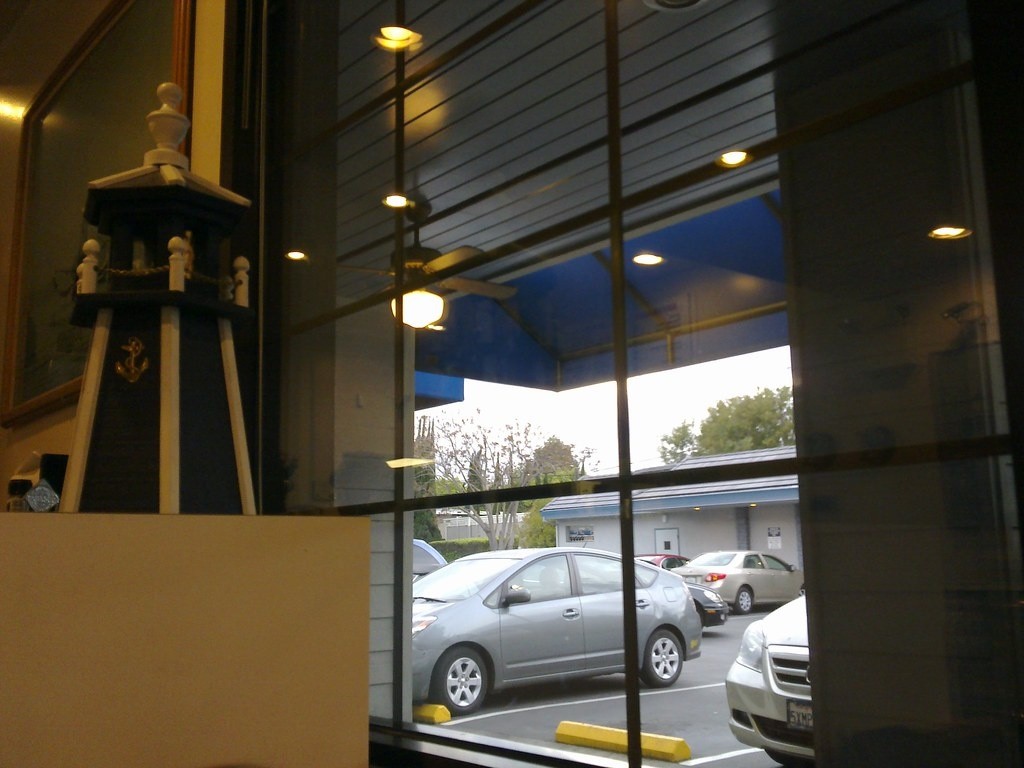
[{"x1": 336, "y1": 165, "x2": 519, "y2": 304}]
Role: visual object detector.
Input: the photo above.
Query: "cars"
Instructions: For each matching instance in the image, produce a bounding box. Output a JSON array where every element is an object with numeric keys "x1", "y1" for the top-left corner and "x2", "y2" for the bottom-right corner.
[
  {"x1": 667, "y1": 549, "x2": 806, "y2": 616},
  {"x1": 411, "y1": 538, "x2": 703, "y2": 718},
  {"x1": 684, "y1": 580, "x2": 729, "y2": 629},
  {"x1": 605, "y1": 553, "x2": 690, "y2": 585},
  {"x1": 725, "y1": 594, "x2": 816, "y2": 768}
]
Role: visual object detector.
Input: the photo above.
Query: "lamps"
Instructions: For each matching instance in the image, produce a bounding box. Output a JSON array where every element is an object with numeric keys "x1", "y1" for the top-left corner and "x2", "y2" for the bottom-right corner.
[{"x1": 389, "y1": 282, "x2": 447, "y2": 330}]
